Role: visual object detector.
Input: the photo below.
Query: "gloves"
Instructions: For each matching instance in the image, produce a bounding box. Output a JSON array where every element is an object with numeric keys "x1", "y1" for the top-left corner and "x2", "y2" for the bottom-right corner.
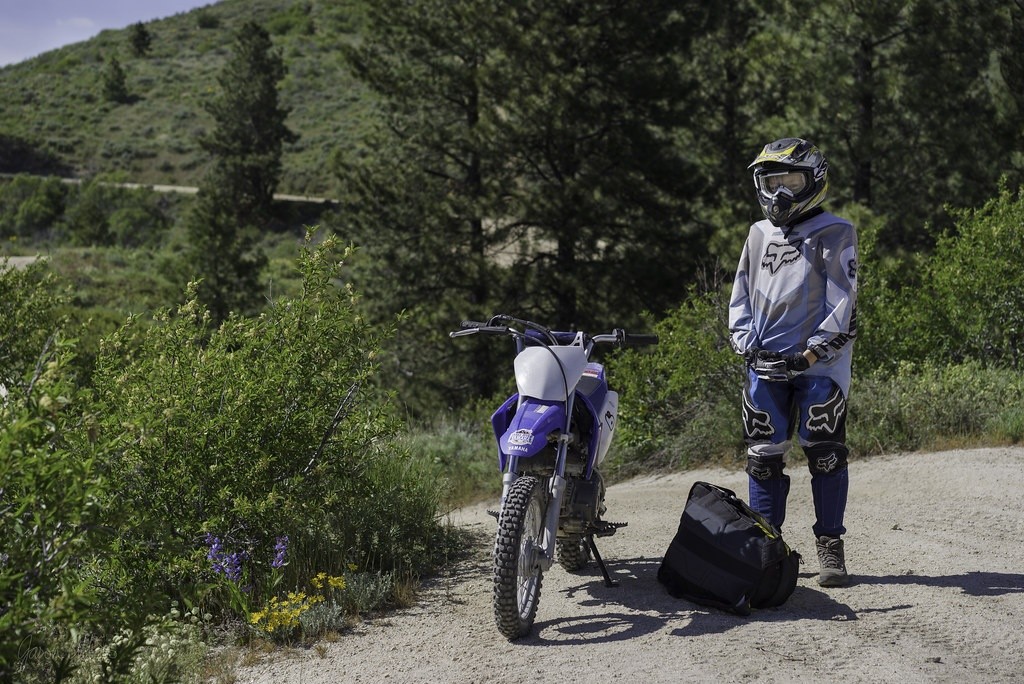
[
  {"x1": 744, "y1": 346, "x2": 789, "y2": 382},
  {"x1": 766, "y1": 352, "x2": 811, "y2": 380}
]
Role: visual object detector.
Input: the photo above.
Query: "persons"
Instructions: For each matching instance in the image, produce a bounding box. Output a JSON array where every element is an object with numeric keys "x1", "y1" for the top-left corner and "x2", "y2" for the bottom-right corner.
[{"x1": 728, "y1": 137, "x2": 860, "y2": 589}]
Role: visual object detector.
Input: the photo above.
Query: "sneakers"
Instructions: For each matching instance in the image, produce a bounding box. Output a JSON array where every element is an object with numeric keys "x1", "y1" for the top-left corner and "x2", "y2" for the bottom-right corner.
[{"x1": 816, "y1": 536, "x2": 847, "y2": 585}]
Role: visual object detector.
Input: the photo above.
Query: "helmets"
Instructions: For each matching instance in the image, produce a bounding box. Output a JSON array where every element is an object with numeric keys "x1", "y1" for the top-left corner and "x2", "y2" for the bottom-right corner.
[{"x1": 747, "y1": 137, "x2": 829, "y2": 226}]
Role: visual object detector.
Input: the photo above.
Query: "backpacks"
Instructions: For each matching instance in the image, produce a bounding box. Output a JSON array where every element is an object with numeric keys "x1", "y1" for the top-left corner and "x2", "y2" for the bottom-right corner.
[{"x1": 658, "y1": 481, "x2": 800, "y2": 617}]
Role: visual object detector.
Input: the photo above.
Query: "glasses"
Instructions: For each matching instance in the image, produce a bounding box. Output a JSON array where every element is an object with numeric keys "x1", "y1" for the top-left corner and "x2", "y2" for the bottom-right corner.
[{"x1": 758, "y1": 172, "x2": 810, "y2": 197}]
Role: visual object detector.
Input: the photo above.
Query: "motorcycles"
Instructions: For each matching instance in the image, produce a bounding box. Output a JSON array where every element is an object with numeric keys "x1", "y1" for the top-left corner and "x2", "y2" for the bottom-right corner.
[{"x1": 446, "y1": 313, "x2": 660, "y2": 639}]
